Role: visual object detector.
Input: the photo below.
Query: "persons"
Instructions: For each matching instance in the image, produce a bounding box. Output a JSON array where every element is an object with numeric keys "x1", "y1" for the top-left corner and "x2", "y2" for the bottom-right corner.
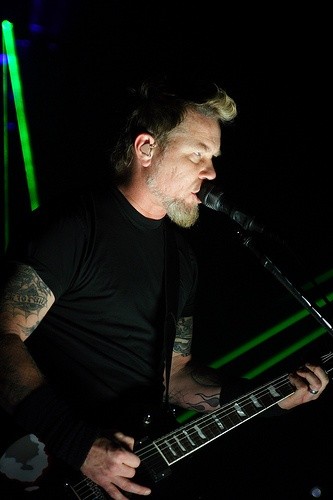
[{"x1": 0, "y1": 63, "x2": 330, "y2": 500}]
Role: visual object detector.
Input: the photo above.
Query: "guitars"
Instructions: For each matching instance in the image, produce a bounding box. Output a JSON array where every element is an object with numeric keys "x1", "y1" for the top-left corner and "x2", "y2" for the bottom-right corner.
[{"x1": 60, "y1": 346, "x2": 333, "y2": 500}]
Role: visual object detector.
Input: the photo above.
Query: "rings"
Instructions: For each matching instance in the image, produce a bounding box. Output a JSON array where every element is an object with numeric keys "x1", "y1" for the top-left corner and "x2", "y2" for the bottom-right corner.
[{"x1": 307, "y1": 386, "x2": 319, "y2": 396}]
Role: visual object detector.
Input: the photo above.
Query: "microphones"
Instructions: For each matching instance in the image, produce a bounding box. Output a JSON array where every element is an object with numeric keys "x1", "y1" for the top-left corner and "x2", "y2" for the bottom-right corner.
[{"x1": 199, "y1": 182, "x2": 263, "y2": 233}]
